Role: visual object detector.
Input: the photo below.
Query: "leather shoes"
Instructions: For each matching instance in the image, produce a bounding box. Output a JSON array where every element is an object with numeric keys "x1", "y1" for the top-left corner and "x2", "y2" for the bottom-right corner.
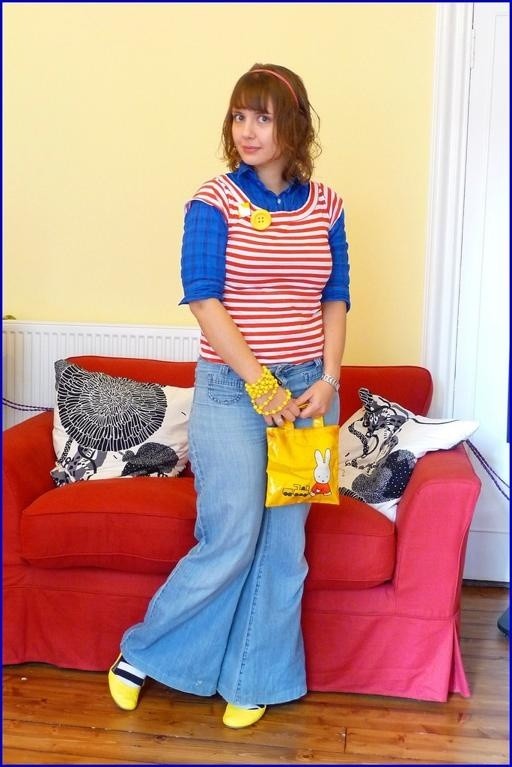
[
  {"x1": 222, "y1": 702, "x2": 267, "y2": 729},
  {"x1": 108, "y1": 651, "x2": 147, "y2": 712}
]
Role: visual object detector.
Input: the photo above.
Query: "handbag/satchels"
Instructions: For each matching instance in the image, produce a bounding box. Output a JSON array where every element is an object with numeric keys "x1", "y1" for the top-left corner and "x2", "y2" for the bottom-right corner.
[{"x1": 264, "y1": 424, "x2": 340, "y2": 508}]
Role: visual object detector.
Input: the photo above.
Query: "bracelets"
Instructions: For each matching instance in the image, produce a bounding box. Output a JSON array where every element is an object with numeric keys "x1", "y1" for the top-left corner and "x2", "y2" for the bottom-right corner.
[
  {"x1": 243, "y1": 364, "x2": 292, "y2": 416},
  {"x1": 319, "y1": 372, "x2": 341, "y2": 392}
]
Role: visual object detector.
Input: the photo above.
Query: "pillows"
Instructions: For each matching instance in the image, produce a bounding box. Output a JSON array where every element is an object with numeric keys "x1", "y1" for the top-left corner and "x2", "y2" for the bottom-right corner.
[
  {"x1": 50, "y1": 358, "x2": 196, "y2": 487},
  {"x1": 339, "y1": 387, "x2": 480, "y2": 523}
]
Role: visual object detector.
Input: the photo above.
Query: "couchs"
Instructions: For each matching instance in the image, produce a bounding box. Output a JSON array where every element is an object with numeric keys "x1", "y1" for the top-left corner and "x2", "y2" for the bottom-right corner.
[{"x1": 2, "y1": 355, "x2": 482, "y2": 700}]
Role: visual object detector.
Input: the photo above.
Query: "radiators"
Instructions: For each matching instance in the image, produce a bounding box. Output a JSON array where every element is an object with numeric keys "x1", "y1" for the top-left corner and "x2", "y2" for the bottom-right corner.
[{"x1": 3, "y1": 319, "x2": 201, "y2": 432}]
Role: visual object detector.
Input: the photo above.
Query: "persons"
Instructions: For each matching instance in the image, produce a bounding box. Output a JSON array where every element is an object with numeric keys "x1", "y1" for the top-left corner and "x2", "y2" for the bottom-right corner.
[{"x1": 105, "y1": 61, "x2": 353, "y2": 729}]
